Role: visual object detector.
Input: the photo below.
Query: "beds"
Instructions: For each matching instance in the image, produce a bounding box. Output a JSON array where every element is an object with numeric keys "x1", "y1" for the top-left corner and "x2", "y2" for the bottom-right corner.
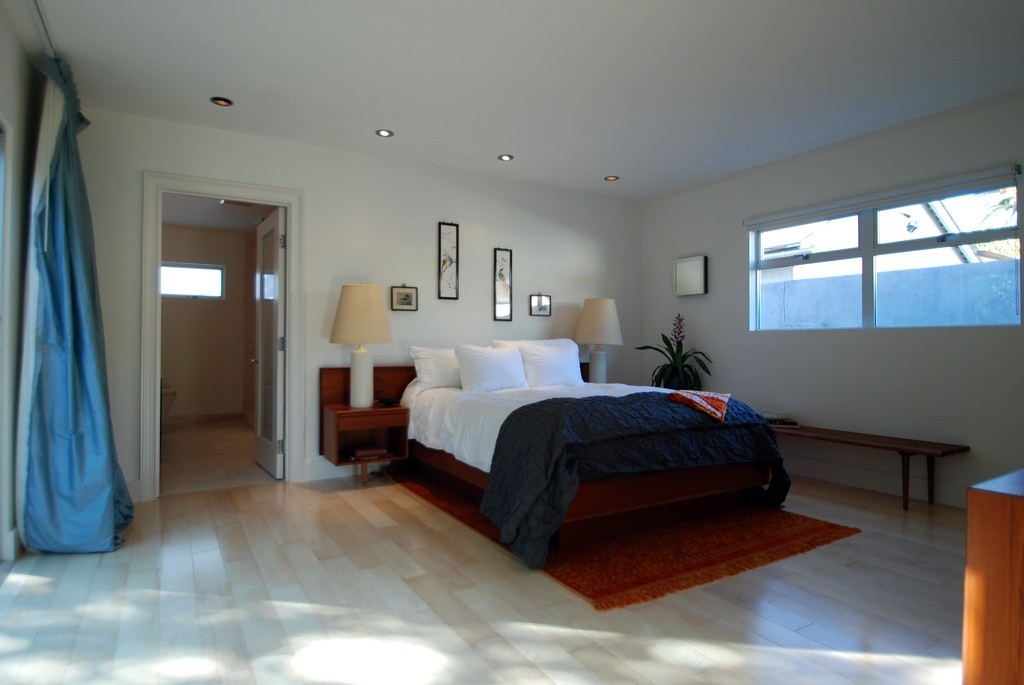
[{"x1": 319, "y1": 365, "x2": 791, "y2": 568}]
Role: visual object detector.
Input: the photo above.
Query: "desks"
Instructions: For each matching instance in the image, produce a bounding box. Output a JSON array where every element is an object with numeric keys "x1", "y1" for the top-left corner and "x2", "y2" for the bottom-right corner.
[{"x1": 958, "y1": 469, "x2": 1024, "y2": 685}]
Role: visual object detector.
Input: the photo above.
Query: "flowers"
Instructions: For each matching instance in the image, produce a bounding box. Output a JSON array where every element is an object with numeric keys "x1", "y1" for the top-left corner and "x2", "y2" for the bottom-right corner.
[{"x1": 633, "y1": 311, "x2": 712, "y2": 391}]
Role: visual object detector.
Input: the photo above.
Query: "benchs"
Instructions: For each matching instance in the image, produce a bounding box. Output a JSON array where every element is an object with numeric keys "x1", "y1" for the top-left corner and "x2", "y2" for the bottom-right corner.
[{"x1": 772, "y1": 425, "x2": 970, "y2": 510}]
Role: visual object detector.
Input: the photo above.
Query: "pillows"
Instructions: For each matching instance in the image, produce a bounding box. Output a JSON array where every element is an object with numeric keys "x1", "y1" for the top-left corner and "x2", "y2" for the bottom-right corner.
[
  {"x1": 454, "y1": 344, "x2": 528, "y2": 392},
  {"x1": 493, "y1": 338, "x2": 584, "y2": 388},
  {"x1": 407, "y1": 346, "x2": 461, "y2": 395}
]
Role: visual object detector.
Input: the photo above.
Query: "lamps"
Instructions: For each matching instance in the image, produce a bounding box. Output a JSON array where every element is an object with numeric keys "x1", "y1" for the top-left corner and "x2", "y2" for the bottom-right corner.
[
  {"x1": 329, "y1": 283, "x2": 393, "y2": 409},
  {"x1": 574, "y1": 299, "x2": 623, "y2": 383}
]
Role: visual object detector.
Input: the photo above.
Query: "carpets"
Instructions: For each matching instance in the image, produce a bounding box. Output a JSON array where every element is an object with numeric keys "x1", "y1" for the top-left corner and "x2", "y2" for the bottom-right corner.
[{"x1": 377, "y1": 464, "x2": 862, "y2": 614}]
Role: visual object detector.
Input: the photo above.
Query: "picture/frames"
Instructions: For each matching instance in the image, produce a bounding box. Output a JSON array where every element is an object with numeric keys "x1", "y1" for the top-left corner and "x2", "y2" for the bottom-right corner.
[
  {"x1": 673, "y1": 256, "x2": 709, "y2": 296},
  {"x1": 391, "y1": 286, "x2": 418, "y2": 311},
  {"x1": 437, "y1": 222, "x2": 459, "y2": 299},
  {"x1": 493, "y1": 248, "x2": 513, "y2": 321},
  {"x1": 530, "y1": 294, "x2": 552, "y2": 316}
]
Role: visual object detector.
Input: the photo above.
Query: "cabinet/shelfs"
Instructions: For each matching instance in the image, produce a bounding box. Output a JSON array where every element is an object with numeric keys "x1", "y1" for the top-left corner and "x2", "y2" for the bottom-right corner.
[{"x1": 321, "y1": 404, "x2": 411, "y2": 484}]
